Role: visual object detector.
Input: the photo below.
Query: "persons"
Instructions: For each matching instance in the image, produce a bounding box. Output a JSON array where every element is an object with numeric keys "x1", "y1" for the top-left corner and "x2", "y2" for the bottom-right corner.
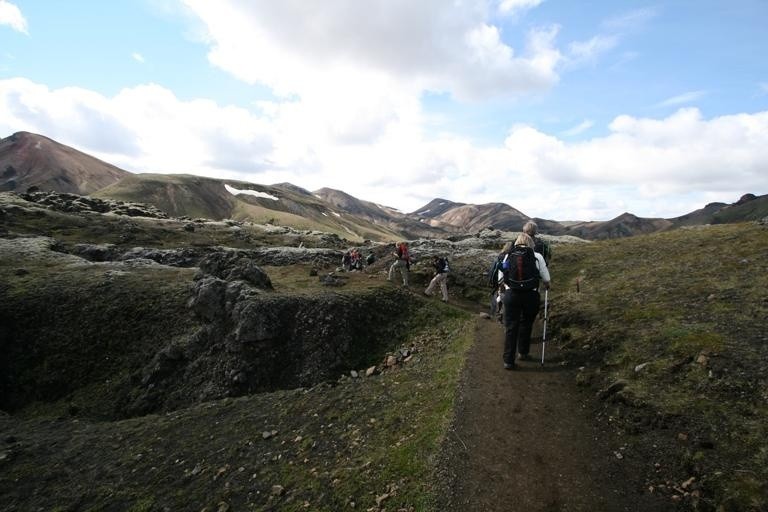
[
  {"x1": 387, "y1": 240, "x2": 410, "y2": 288},
  {"x1": 498, "y1": 233, "x2": 552, "y2": 369},
  {"x1": 498, "y1": 220, "x2": 552, "y2": 340},
  {"x1": 424, "y1": 255, "x2": 450, "y2": 303},
  {"x1": 487, "y1": 242, "x2": 515, "y2": 321},
  {"x1": 342, "y1": 247, "x2": 376, "y2": 270},
  {"x1": 299, "y1": 241, "x2": 305, "y2": 249}
]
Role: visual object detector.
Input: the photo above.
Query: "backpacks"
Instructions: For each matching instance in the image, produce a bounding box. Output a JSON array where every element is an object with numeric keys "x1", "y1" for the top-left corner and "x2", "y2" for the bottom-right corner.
[
  {"x1": 501, "y1": 246, "x2": 539, "y2": 290},
  {"x1": 400, "y1": 243, "x2": 409, "y2": 260},
  {"x1": 440, "y1": 256, "x2": 450, "y2": 272}
]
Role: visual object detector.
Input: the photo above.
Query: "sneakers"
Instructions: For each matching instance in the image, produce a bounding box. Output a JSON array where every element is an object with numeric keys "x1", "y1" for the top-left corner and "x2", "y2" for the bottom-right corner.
[{"x1": 504, "y1": 353, "x2": 526, "y2": 370}]
